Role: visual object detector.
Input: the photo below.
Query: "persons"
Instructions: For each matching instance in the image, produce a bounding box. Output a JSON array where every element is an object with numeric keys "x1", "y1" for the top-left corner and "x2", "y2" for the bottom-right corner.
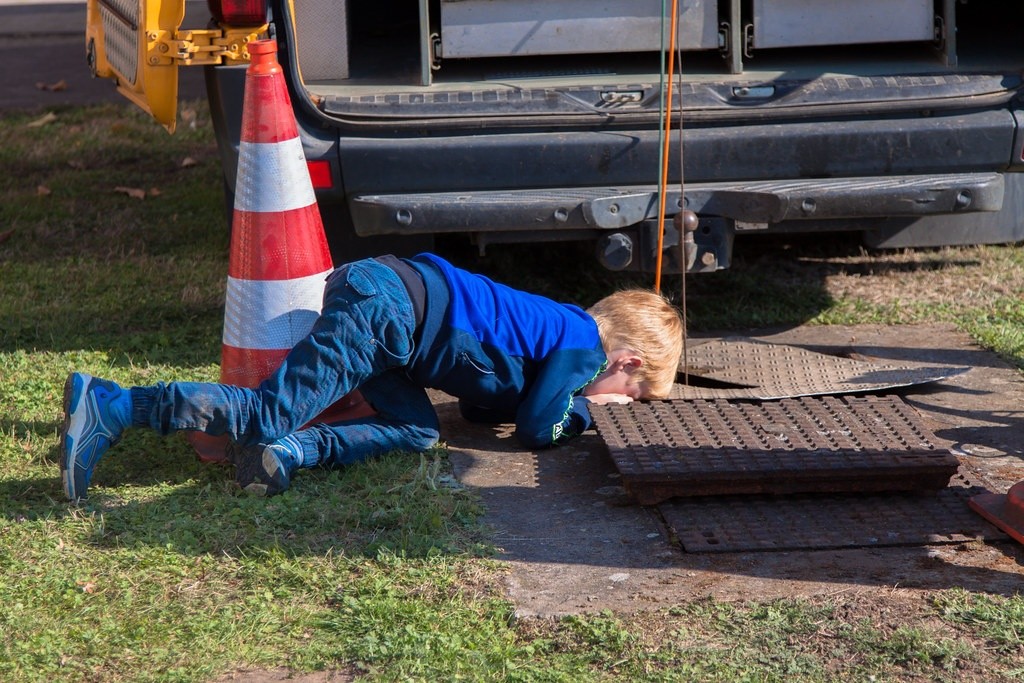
[{"x1": 61, "y1": 254, "x2": 682, "y2": 500}]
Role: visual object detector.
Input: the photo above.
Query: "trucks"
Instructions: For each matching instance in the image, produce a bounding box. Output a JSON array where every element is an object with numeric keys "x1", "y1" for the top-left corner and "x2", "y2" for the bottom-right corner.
[{"x1": 83, "y1": 1, "x2": 1024, "y2": 278}]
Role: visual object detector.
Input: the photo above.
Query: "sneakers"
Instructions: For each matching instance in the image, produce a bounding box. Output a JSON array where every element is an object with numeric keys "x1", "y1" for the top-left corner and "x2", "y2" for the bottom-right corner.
[
  {"x1": 61, "y1": 373, "x2": 122, "y2": 499},
  {"x1": 233, "y1": 435, "x2": 301, "y2": 493}
]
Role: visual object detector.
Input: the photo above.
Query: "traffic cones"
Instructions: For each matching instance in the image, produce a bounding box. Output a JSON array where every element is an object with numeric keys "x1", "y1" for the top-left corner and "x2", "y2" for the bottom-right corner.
[{"x1": 178, "y1": 36, "x2": 378, "y2": 470}]
